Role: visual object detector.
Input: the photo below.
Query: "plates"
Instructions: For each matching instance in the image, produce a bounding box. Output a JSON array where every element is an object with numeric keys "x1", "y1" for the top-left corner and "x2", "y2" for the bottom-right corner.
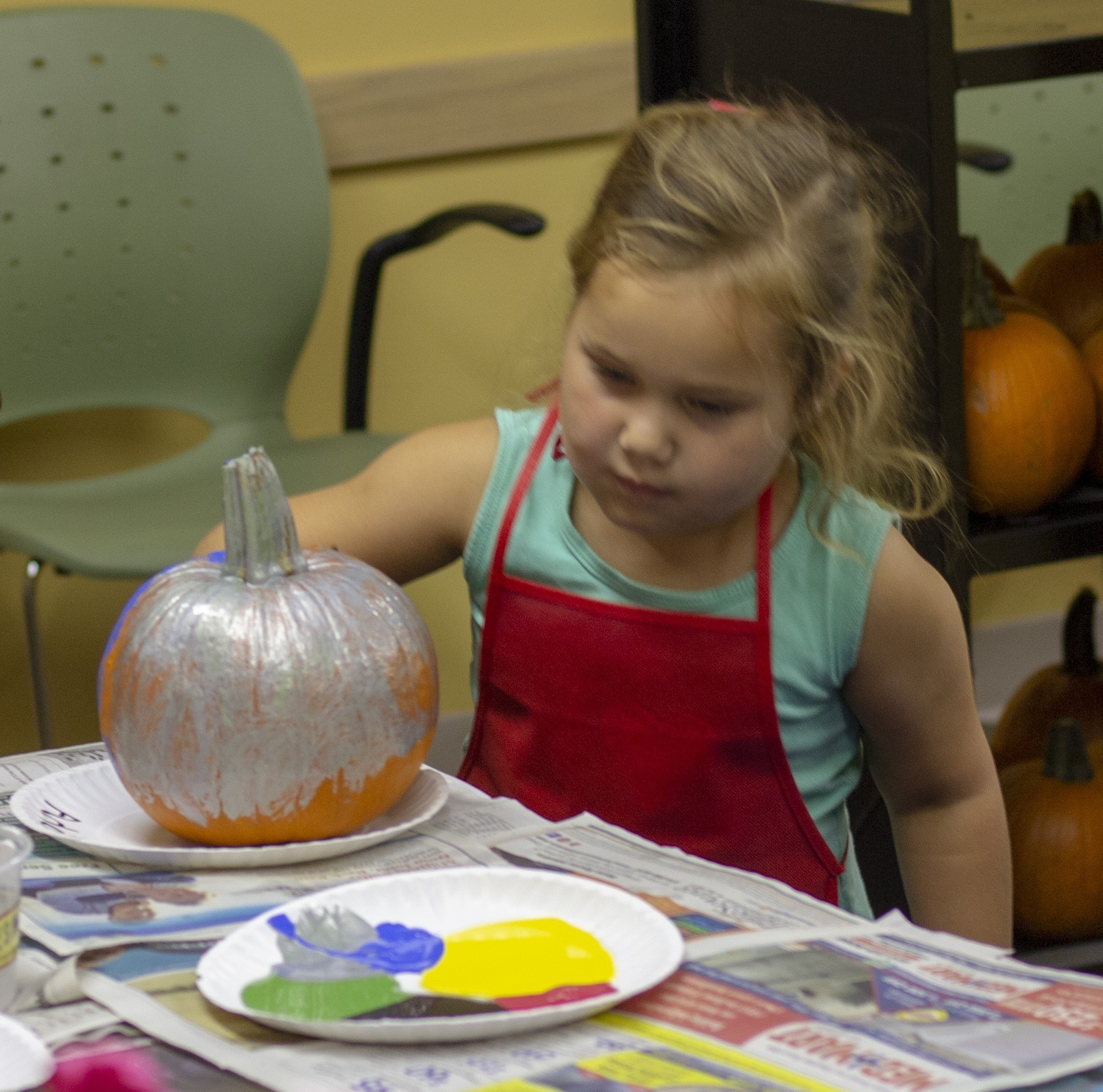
[
  {"x1": 9, "y1": 756, "x2": 450, "y2": 868},
  {"x1": 194, "y1": 865, "x2": 684, "y2": 1043}
]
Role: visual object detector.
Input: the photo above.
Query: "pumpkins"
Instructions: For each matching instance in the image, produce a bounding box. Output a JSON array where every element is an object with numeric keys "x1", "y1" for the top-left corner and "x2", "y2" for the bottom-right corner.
[
  {"x1": 96, "y1": 447, "x2": 443, "y2": 845},
  {"x1": 990, "y1": 584, "x2": 1103, "y2": 943},
  {"x1": 962, "y1": 187, "x2": 1102, "y2": 517}
]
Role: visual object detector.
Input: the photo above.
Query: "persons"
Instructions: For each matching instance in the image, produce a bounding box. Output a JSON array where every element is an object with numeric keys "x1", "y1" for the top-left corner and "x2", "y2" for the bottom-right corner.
[
  {"x1": 188, "y1": 96, "x2": 1019, "y2": 963},
  {"x1": 25, "y1": 880, "x2": 207, "y2": 927}
]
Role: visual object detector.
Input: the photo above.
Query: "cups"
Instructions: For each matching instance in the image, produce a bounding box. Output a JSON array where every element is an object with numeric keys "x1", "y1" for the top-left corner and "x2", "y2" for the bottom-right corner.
[{"x1": 0, "y1": 824, "x2": 32, "y2": 1012}]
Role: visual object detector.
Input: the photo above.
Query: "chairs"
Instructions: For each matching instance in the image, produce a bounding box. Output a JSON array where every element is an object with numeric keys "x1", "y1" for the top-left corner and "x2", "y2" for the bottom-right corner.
[{"x1": 0, "y1": 5, "x2": 550, "y2": 751}]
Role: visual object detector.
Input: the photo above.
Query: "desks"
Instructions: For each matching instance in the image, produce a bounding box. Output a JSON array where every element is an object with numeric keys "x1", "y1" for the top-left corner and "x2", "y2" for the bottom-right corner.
[{"x1": 0, "y1": 742, "x2": 1103, "y2": 1092}]
